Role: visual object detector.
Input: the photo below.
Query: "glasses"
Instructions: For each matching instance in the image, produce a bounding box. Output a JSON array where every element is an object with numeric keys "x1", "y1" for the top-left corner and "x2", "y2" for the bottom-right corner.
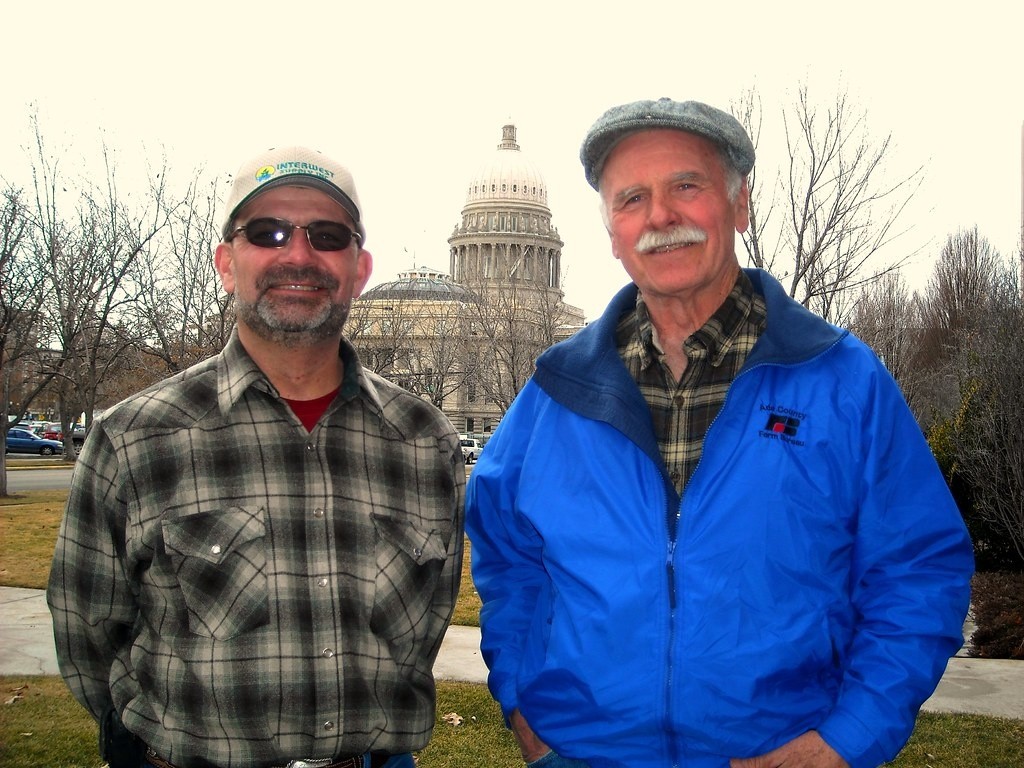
[{"x1": 225, "y1": 218, "x2": 364, "y2": 253}]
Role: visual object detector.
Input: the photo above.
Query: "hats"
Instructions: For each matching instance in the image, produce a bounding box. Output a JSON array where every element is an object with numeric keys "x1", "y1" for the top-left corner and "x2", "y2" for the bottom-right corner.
[
  {"x1": 580, "y1": 97, "x2": 756, "y2": 192},
  {"x1": 220, "y1": 144, "x2": 364, "y2": 248}
]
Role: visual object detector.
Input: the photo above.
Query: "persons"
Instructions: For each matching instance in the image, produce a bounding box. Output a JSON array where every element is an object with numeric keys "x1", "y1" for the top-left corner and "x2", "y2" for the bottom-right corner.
[
  {"x1": 464, "y1": 97, "x2": 977, "y2": 768},
  {"x1": 47, "y1": 148, "x2": 465, "y2": 768}
]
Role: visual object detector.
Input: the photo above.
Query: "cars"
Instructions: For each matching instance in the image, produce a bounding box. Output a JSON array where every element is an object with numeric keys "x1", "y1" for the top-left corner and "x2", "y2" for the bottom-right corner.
[
  {"x1": 13, "y1": 421, "x2": 88, "y2": 454},
  {"x1": 461, "y1": 438, "x2": 484, "y2": 464},
  {"x1": 6, "y1": 428, "x2": 64, "y2": 455}
]
